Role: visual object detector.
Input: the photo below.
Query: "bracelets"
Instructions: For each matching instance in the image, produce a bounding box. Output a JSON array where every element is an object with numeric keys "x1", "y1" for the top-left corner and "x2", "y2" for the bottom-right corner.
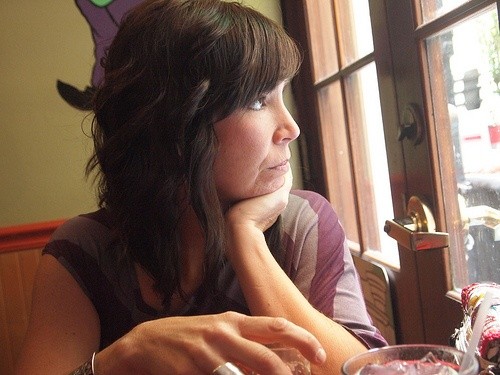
[{"x1": 71, "y1": 352, "x2": 95, "y2": 375}]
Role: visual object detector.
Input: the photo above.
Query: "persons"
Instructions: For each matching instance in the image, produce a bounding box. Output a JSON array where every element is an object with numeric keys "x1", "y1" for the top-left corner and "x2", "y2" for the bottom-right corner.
[{"x1": 8, "y1": 0, "x2": 390, "y2": 375}]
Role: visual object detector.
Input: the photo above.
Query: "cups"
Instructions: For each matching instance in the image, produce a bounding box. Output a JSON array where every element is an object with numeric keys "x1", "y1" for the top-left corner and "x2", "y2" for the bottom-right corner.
[
  {"x1": 228, "y1": 342, "x2": 312, "y2": 375},
  {"x1": 341, "y1": 342, "x2": 479, "y2": 375}
]
